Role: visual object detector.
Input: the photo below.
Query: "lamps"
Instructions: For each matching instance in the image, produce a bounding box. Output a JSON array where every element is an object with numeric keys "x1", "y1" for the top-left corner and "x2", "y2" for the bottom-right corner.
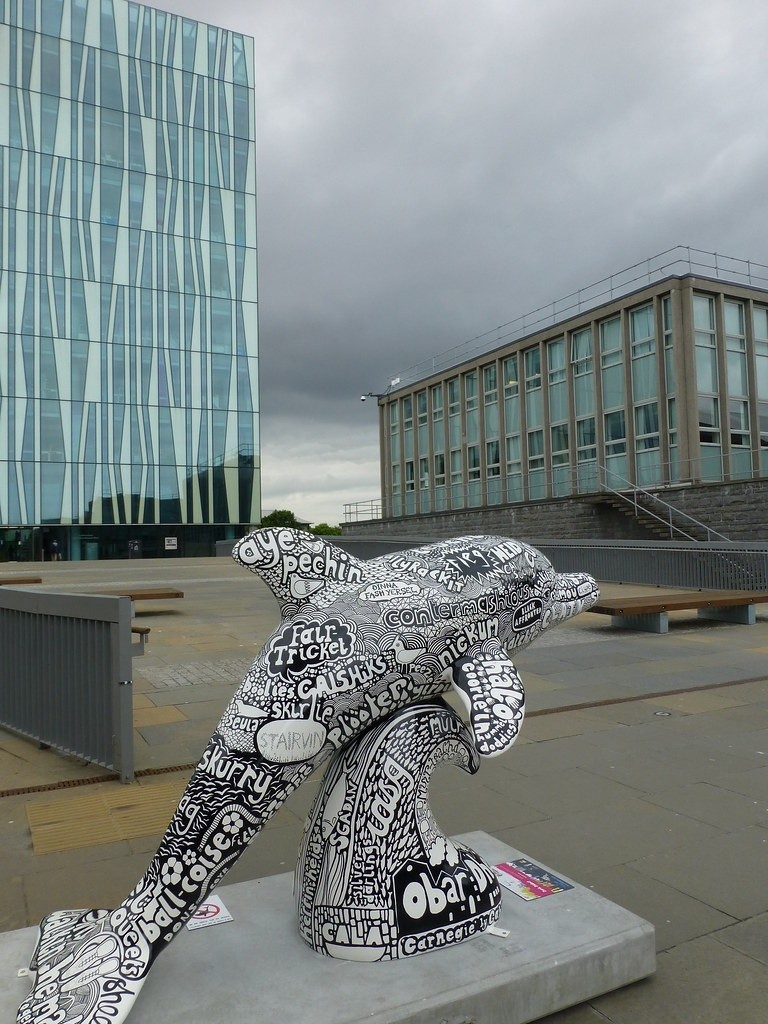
[{"x1": 390, "y1": 378, "x2": 417, "y2": 386}]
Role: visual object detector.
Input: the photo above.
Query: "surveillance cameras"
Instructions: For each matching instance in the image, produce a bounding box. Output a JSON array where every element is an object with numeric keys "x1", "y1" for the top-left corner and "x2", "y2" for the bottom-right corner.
[{"x1": 361, "y1": 396, "x2": 366, "y2": 401}]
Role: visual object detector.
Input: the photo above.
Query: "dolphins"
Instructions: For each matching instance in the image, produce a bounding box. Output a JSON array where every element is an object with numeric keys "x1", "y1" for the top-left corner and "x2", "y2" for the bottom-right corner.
[{"x1": 16, "y1": 527, "x2": 599, "y2": 1024}]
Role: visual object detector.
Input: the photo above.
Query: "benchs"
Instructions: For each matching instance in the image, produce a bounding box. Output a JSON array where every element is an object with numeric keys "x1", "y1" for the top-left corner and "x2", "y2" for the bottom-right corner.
[
  {"x1": 0, "y1": 575, "x2": 43, "y2": 586},
  {"x1": 585, "y1": 589, "x2": 768, "y2": 634},
  {"x1": 72, "y1": 587, "x2": 184, "y2": 618}
]
